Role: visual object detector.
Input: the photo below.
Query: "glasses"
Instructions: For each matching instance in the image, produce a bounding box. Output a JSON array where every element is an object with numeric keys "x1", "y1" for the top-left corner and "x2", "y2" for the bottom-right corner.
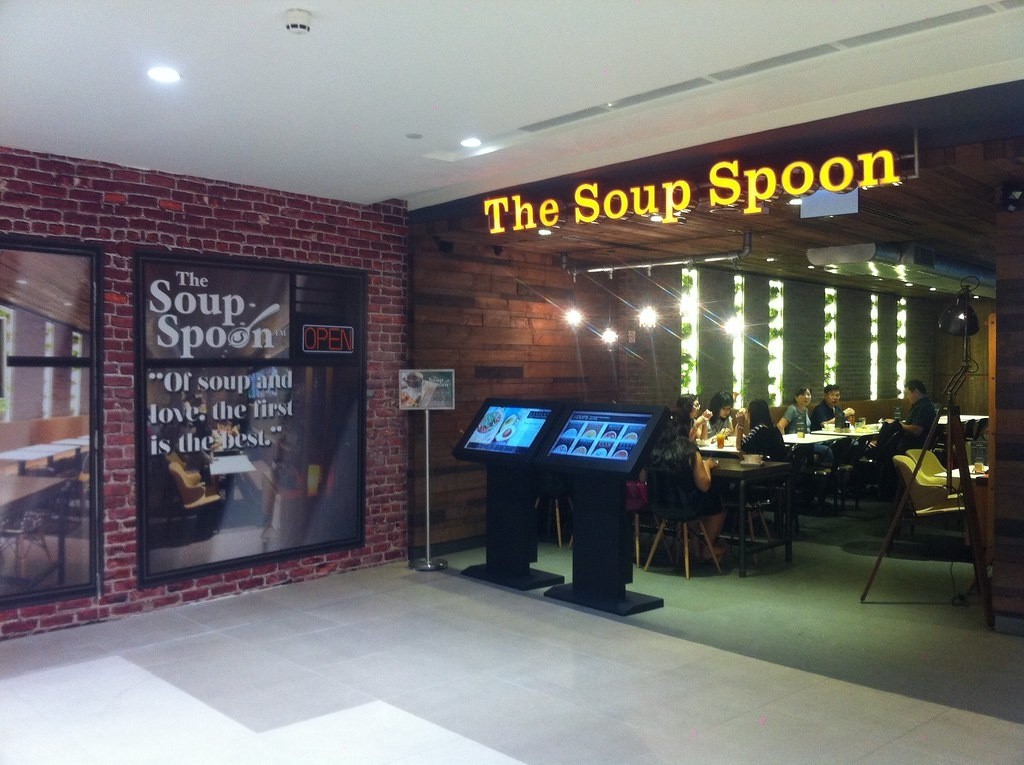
[{"x1": 692, "y1": 403, "x2": 702, "y2": 411}]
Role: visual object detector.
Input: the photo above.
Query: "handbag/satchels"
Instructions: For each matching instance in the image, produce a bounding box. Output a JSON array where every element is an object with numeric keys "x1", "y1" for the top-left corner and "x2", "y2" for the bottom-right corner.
[
  {"x1": 625, "y1": 482, "x2": 650, "y2": 512},
  {"x1": 876, "y1": 419, "x2": 902, "y2": 452}
]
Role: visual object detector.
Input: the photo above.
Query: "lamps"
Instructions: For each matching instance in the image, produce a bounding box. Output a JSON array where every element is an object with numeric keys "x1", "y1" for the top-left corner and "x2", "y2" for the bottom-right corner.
[{"x1": 938, "y1": 276, "x2": 980, "y2": 402}]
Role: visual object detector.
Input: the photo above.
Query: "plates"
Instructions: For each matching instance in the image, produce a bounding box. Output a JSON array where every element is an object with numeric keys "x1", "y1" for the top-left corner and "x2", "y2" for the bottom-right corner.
[
  {"x1": 554, "y1": 445, "x2": 567, "y2": 454},
  {"x1": 573, "y1": 447, "x2": 586, "y2": 455},
  {"x1": 740, "y1": 461, "x2": 764, "y2": 465},
  {"x1": 623, "y1": 433, "x2": 637, "y2": 440},
  {"x1": 581, "y1": 430, "x2": 597, "y2": 439},
  {"x1": 502, "y1": 415, "x2": 516, "y2": 427},
  {"x1": 496, "y1": 425, "x2": 515, "y2": 441},
  {"x1": 602, "y1": 431, "x2": 616, "y2": 439},
  {"x1": 564, "y1": 429, "x2": 577, "y2": 437},
  {"x1": 477, "y1": 412, "x2": 503, "y2": 435},
  {"x1": 593, "y1": 449, "x2": 606, "y2": 457},
  {"x1": 613, "y1": 450, "x2": 628, "y2": 459}
]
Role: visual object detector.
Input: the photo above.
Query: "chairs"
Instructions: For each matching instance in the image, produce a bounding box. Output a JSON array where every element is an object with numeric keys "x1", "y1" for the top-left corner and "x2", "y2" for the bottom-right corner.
[
  {"x1": 535, "y1": 418, "x2": 989, "y2": 580},
  {"x1": 163, "y1": 451, "x2": 221, "y2": 538}
]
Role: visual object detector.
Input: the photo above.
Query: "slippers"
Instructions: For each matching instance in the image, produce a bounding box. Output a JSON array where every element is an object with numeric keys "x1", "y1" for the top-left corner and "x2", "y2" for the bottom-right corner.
[{"x1": 712, "y1": 543, "x2": 727, "y2": 564}]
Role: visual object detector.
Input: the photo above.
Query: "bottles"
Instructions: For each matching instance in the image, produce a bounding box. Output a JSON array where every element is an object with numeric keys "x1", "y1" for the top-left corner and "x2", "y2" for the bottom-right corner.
[
  {"x1": 894, "y1": 408, "x2": 900, "y2": 421},
  {"x1": 935, "y1": 405, "x2": 939, "y2": 413},
  {"x1": 797, "y1": 417, "x2": 804, "y2": 438},
  {"x1": 845, "y1": 420, "x2": 849, "y2": 432}
]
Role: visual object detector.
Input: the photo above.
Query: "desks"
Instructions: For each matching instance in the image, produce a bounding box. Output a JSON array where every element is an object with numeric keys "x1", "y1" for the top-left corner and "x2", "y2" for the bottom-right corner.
[
  {"x1": 903, "y1": 415, "x2": 989, "y2": 425},
  {"x1": 782, "y1": 433, "x2": 847, "y2": 513},
  {"x1": 699, "y1": 439, "x2": 740, "y2": 458},
  {"x1": 701, "y1": 456, "x2": 792, "y2": 577},
  {"x1": 934, "y1": 464, "x2": 989, "y2": 547},
  {"x1": 209, "y1": 455, "x2": 256, "y2": 516},
  {"x1": 0, "y1": 434, "x2": 90, "y2": 597},
  {"x1": 811, "y1": 423, "x2": 882, "y2": 499}
]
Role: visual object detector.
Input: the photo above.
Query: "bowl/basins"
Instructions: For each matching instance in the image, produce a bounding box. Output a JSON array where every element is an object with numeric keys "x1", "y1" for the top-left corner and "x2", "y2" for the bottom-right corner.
[
  {"x1": 744, "y1": 454, "x2": 763, "y2": 463},
  {"x1": 728, "y1": 437, "x2": 736, "y2": 446},
  {"x1": 867, "y1": 425, "x2": 878, "y2": 430}
]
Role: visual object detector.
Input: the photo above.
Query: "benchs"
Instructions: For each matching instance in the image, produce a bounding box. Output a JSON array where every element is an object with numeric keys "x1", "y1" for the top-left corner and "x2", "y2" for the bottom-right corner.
[
  {"x1": 0, "y1": 414, "x2": 90, "y2": 475},
  {"x1": 671, "y1": 398, "x2": 912, "y2": 435},
  {"x1": 236, "y1": 425, "x2": 297, "y2": 511}
]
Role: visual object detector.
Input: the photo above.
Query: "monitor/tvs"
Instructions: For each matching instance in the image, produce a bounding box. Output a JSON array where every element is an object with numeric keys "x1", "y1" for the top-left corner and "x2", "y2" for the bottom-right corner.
[
  {"x1": 463, "y1": 405, "x2": 551, "y2": 455},
  {"x1": 550, "y1": 410, "x2": 652, "y2": 460}
]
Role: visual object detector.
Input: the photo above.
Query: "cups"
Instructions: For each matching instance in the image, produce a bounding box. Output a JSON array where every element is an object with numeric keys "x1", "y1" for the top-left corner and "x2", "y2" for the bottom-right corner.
[
  {"x1": 828, "y1": 424, "x2": 835, "y2": 429},
  {"x1": 403, "y1": 373, "x2": 423, "y2": 392},
  {"x1": 717, "y1": 434, "x2": 724, "y2": 449},
  {"x1": 855, "y1": 420, "x2": 862, "y2": 428},
  {"x1": 860, "y1": 418, "x2": 865, "y2": 426},
  {"x1": 975, "y1": 463, "x2": 983, "y2": 471},
  {"x1": 420, "y1": 380, "x2": 438, "y2": 406},
  {"x1": 849, "y1": 416, "x2": 855, "y2": 426}
]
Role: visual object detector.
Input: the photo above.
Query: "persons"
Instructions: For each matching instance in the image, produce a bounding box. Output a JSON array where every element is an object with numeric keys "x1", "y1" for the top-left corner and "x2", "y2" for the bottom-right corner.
[
  {"x1": 708, "y1": 391, "x2": 740, "y2": 453},
  {"x1": 735, "y1": 400, "x2": 785, "y2": 462},
  {"x1": 638, "y1": 408, "x2": 727, "y2": 560},
  {"x1": 671, "y1": 395, "x2": 713, "y2": 442},
  {"x1": 864, "y1": 380, "x2": 936, "y2": 502},
  {"x1": 776, "y1": 387, "x2": 834, "y2": 470},
  {"x1": 811, "y1": 384, "x2": 876, "y2": 471}
]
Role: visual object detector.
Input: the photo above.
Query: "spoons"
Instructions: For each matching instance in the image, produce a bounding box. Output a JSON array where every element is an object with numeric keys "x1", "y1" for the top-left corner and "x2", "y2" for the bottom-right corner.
[{"x1": 228, "y1": 304, "x2": 280, "y2": 348}]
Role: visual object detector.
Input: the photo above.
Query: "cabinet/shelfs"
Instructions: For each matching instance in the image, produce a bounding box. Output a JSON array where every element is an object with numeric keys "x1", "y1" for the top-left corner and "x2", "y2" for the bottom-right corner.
[{"x1": 259, "y1": 472, "x2": 317, "y2": 538}]
